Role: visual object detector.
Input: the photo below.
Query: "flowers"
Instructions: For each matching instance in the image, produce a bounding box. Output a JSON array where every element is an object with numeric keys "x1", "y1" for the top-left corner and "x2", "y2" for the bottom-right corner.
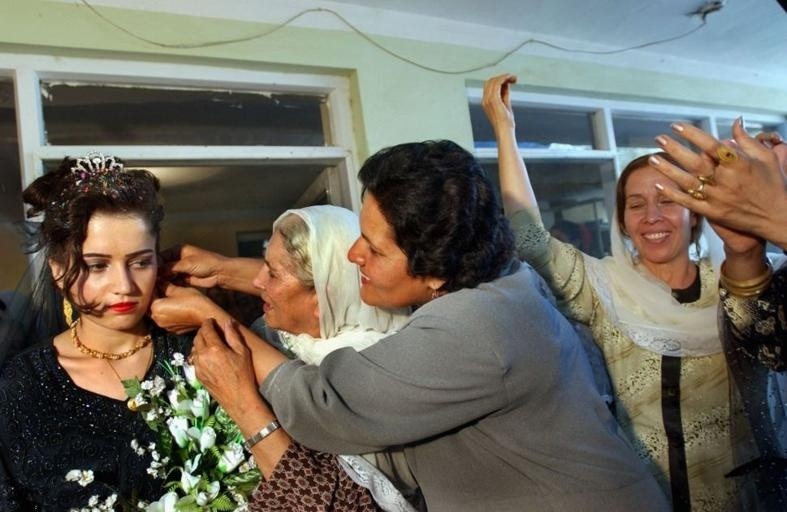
[{"x1": 64, "y1": 347, "x2": 261, "y2": 512}]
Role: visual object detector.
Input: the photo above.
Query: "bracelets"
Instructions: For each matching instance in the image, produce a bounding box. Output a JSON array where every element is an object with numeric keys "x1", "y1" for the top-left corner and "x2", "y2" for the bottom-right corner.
[
  {"x1": 246, "y1": 420, "x2": 279, "y2": 449},
  {"x1": 720, "y1": 253, "x2": 774, "y2": 297}
]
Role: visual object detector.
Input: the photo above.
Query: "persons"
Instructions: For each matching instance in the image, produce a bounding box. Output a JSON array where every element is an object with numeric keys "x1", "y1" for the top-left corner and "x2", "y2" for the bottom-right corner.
[
  {"x1": 0, "y1": 155, "x2": 253, "y2": 512},
  {"x1": 147, "y1": 137, "x2": 675, "y2": 512},
  {"x1": 189, "y1": 205, "x2": 426, "y2": 512},
  {"x1": 648, "y1": 114, "x2": 787, "y2": 373},
  {"x1": 1, "y1": 290, "x2": 61, "y2": 360},
  {"x1": 481, "y1": 71, "x2": 787, "y2": 512}
]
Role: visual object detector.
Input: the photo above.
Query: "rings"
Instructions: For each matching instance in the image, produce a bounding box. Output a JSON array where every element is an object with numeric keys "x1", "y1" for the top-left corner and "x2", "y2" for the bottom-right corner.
[
  {"x1": 687, "y1": 183, "x2": 705, "y2": 200},
  {"x1": 696, "y1": 174, "x2": 716, "y2": 187},
  {"x1": 714, "y1": 143, "x2": 739, "y2": 164}
]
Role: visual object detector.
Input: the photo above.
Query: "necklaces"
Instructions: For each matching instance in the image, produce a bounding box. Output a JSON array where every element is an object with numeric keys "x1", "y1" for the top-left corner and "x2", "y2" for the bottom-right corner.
[{"x1": 70, "y1": 317, "x2": 153, "y2": 360}]
